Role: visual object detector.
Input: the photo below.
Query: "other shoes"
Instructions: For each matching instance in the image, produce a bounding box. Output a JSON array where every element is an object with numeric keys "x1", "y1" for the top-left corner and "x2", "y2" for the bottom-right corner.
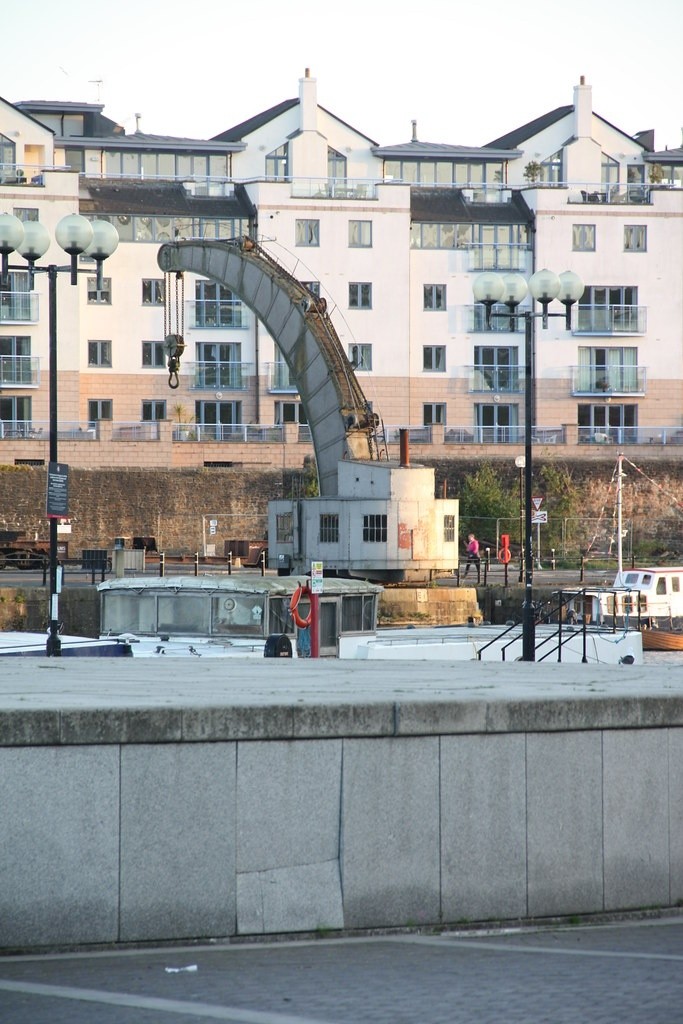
[{"x1": 461, "y1": 575, "x2": 465, "y2": 578}]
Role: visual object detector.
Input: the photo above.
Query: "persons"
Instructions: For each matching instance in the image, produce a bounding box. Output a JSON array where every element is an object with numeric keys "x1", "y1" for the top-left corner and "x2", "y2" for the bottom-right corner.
[{"x1": 461, "y1": 534, "x2": 481, "y2": 578}]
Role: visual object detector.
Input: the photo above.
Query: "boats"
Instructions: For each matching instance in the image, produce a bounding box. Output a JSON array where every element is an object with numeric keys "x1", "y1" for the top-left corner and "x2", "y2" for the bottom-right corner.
[{"x1": 582, "y1": 452, "x2": 683, "y2": 652}]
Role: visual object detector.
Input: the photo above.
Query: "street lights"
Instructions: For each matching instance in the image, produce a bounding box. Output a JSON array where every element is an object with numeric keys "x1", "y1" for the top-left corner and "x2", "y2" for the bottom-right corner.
[
  {"x1": 0, "y1": 207, "x2": 120, "y2": 652},
  {"x1": 471, "y1": 264, "x2": 586, "y2": 662}
]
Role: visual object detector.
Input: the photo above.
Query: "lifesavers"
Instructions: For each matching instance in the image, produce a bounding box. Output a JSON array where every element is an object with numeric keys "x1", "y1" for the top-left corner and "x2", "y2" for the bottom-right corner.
[
  {"x1": 290, "y1": 586, "x2": 312, "y2": 627},
  {"x1": 498, "y1": 547, "x2": 511, "y2": 563}
]
